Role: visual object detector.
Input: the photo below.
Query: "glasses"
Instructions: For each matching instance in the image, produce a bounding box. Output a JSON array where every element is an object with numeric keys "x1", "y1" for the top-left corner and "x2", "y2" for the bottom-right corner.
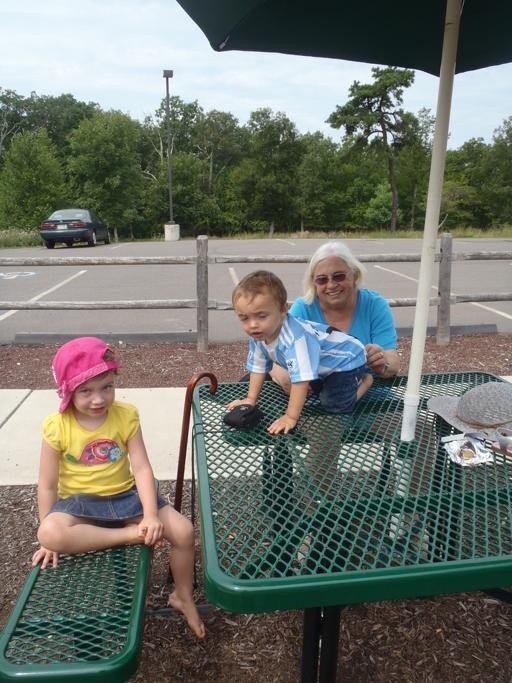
[{"x1": 315, "y1": 273, "x2": 346, "y2": 285}]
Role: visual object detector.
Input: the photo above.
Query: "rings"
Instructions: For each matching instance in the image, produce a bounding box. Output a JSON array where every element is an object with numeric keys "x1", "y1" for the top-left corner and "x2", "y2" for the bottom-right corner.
[{"x1": 377, "y1": 352, "x2": 384, "y2": 359}]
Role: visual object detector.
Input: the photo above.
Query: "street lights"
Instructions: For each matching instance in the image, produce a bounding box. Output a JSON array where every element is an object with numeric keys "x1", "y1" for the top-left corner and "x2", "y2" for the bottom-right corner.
[{"x1": 160, "y1": 69, "x2": 182, "y2": 241}]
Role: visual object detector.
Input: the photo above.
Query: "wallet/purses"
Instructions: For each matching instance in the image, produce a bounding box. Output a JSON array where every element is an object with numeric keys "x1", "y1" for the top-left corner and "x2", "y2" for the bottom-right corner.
[{"x1": 224, "y1": 403, "x2": 266, "y2": 429}]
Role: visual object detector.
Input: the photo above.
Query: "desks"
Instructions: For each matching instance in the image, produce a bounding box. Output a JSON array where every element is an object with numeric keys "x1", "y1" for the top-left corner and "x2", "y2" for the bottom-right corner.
[{"x1": 194, "y1": 371, "x2": 511, "y2": 681}]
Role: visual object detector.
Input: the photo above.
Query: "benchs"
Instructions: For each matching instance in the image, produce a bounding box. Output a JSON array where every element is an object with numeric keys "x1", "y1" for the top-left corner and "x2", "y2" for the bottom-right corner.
[{"x1": 0, "y1": 546, "x2": 150, "y2": 683}]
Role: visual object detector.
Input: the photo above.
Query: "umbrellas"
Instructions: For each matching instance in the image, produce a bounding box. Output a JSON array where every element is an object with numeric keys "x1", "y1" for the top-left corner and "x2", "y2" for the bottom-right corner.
[{"x1": 176, "y1": 0, "x2": 512, "y2": 538}]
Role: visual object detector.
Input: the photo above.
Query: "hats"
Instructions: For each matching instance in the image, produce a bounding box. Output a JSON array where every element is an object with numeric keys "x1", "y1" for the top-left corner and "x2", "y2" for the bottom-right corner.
[
  {"x1": 427, "y1": 382, "x2": 512, "y2": 442},
  {"x1": 51, "y1": 337, "x2": 123, "y2": 414}
]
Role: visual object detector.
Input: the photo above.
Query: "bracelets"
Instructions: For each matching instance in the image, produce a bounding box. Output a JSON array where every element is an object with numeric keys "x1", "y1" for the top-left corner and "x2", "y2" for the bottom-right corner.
[{"x1": 376, "y1": 362, "x2": 390, "y2": 376}]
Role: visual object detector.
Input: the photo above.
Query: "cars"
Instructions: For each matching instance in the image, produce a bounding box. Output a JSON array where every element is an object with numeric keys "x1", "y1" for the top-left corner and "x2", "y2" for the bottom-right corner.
[{"x1": 40, "y1": 209, "x2": 111, "y2": 248}]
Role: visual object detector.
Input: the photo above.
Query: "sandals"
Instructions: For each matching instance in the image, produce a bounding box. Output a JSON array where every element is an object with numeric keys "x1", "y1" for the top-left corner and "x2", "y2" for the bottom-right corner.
[{"x1": 491, "y1": 427, "x2": 512, "y2": 457}]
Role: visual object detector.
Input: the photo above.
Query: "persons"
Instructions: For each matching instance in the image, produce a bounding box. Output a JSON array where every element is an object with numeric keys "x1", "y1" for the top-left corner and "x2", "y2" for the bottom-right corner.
[
  {"x1": 32, "y1": 337, "x2": 206, "y2": 639},
  {"x1": 225, "y1": 271, "x2": 373, "y2": 435},
  {"x1": 239, "y1": 241, "x2": 442, "y2": 562}
]
